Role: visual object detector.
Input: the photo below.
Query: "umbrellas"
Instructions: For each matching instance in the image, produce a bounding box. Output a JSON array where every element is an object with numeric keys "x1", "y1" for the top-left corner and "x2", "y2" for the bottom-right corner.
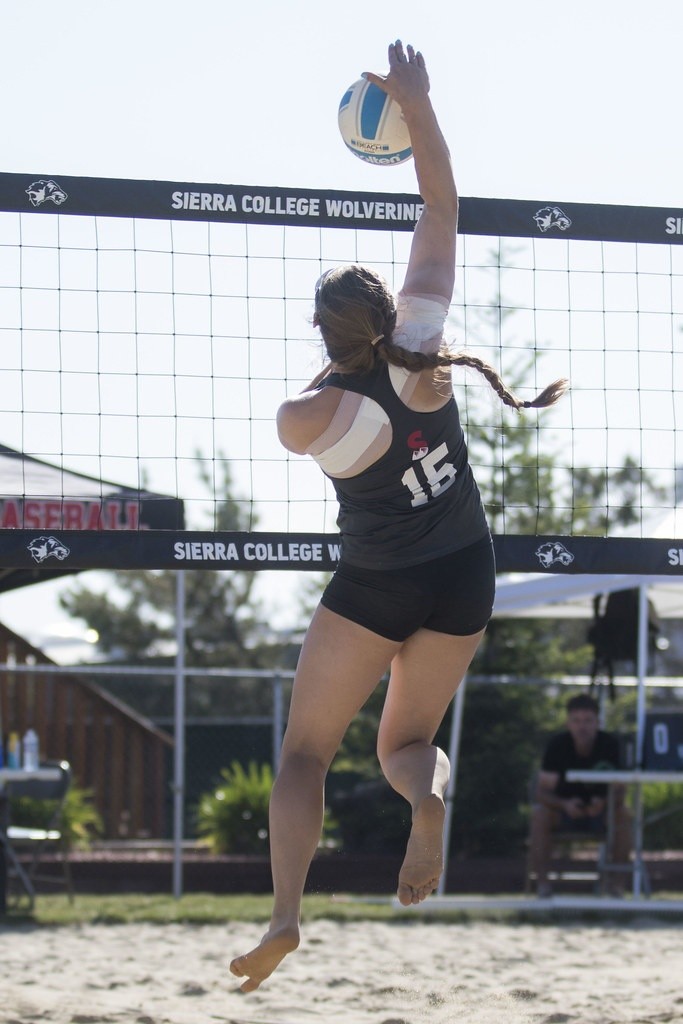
[{"x1": 491, "y1": 507, "x2": 683, "y2": 902}]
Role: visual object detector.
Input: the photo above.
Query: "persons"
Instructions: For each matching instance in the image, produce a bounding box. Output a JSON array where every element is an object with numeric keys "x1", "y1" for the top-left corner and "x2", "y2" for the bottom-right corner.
[
  {"x1": 532, "y1": 694, "x2": 629, "y2": 899},
  {"x1": 229, "y1": 38, "x2": 567, "y2": 991}
]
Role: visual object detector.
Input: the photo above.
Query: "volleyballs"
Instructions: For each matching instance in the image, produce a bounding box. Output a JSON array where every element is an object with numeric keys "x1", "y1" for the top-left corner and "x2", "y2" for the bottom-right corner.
[{"x1": 337, "y1": 75, "x2": 414, "y2": 167}]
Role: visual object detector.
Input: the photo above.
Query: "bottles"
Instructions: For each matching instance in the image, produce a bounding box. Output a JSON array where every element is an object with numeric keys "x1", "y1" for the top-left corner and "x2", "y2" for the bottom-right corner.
[
  {"x1": 7, "y1": 732, "x2": 21, "y2": 768},
  {"x1": 23, "y1": 730, "x2": 39, "y2": 773}
]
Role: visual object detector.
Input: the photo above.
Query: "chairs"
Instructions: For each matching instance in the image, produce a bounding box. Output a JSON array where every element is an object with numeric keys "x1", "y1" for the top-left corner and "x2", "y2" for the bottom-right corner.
[
  {"x1": 518, "y1": 726, "x2": 638, "y2": 896},
  {"x1": 0, "y1": 757, "x2": 75, "y2": 907}
]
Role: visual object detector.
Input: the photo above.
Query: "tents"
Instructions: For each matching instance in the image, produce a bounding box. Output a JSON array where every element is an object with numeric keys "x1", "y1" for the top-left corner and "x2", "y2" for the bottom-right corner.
[
  {"x1": 0, "y1": 439, "x2": 187, "y2": 893},
  {"x1": 268, "y1": 503, "x2": 683, "y2": 900}
]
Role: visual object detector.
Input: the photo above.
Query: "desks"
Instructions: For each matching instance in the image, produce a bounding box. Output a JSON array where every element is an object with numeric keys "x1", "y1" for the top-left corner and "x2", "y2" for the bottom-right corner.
[
  {"x1": 0, "y1": 769, "x2": 66, "y2": 909},
  {"x1": 563, "y1": 765, "x2": 683, "y2": 888}
]
